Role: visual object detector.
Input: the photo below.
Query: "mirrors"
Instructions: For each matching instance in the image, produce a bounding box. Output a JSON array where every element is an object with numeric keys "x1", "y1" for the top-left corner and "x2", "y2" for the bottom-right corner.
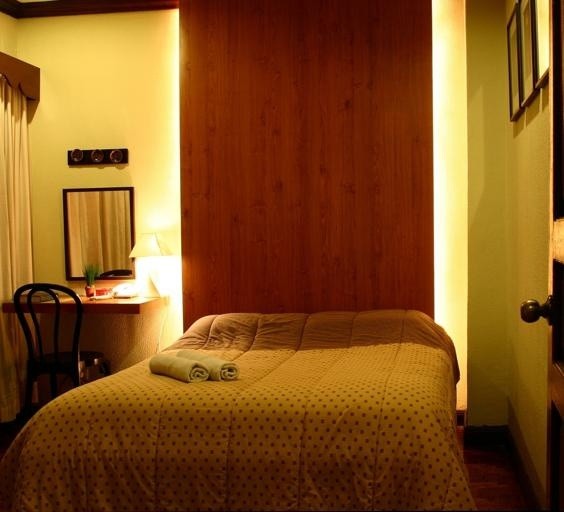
[{"x1": 62, "y1": 186, "x2": 135, "y2": 279}]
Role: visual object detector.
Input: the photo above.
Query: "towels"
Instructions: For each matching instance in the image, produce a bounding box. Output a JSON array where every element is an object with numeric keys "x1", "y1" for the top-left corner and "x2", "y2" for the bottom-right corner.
[{"x1": 149, "y1": 348, "x2": 238, "y2": 384}]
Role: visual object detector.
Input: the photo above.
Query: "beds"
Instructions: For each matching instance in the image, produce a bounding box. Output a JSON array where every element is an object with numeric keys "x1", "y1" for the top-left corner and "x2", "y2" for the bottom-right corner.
[{"x1": 3, "y1": 310, "x2": 459, "y2": 511}]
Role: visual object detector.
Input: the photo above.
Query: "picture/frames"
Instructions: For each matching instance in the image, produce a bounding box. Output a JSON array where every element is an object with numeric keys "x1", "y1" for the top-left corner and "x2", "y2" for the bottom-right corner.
[{"x1": 507, "y1": 0, "x2": 552, "y2": 121}]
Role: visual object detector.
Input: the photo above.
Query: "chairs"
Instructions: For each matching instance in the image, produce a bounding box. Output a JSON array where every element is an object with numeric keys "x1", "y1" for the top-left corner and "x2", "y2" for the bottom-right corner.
[{"x1": 12, "y1": 281, "x2": 107, "y2": 432}]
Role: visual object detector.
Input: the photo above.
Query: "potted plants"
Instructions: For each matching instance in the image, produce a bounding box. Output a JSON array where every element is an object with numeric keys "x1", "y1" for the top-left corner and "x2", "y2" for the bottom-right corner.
[{"x1": 82, "y1": 265, "x2": 100, "y2": 297}]
